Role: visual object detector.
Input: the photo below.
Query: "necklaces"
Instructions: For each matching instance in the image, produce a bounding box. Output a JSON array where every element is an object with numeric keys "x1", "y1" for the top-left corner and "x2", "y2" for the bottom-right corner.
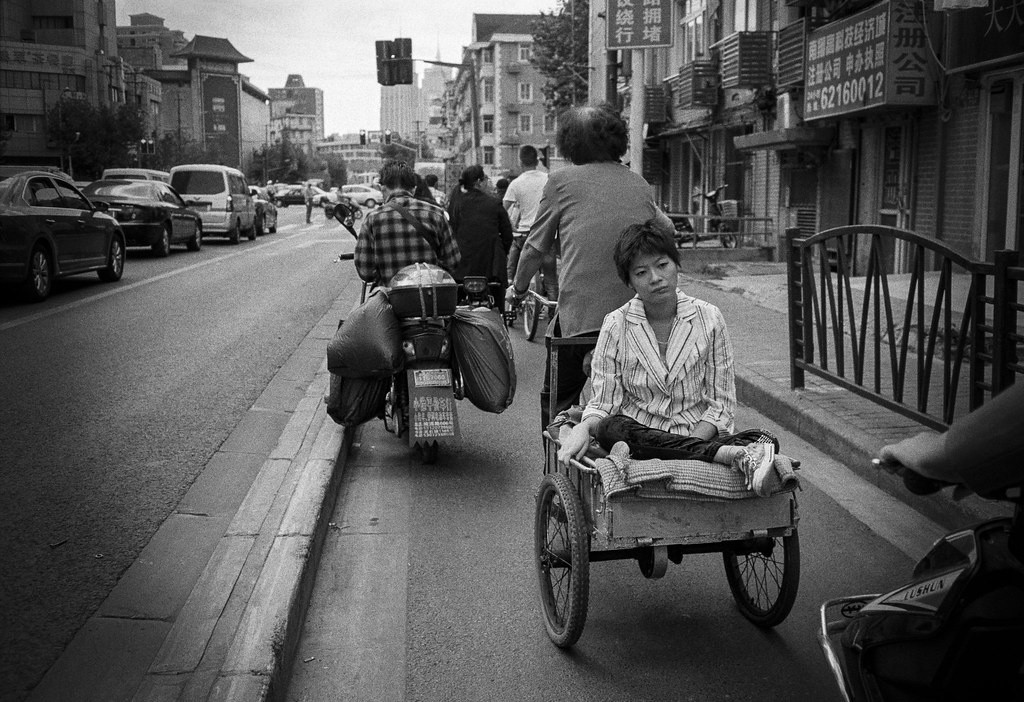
[{"x1": 657, "y1": 341, "x2": 668, "y2": 345}]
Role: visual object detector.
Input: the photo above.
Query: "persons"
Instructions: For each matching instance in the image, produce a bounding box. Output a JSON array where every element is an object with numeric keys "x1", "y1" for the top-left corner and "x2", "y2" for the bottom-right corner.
[
  {"x1": 354, "y1": 159, "x2": 462, "y2": 283},
  {"x1": 556, "y1": 220, "x2": 775, "y2": 498},
  {"x1": 503, "y1": 145, "x2": 558, "y2": 319},
  {"x1": 336, "y1": 185, "x2": 350, "y2": 203},
  {"x1": 504, "y1": 102, "x2": 675, "y2": 523},
  {"x1": 412, "y1": 165, "x2": 512, "y2": 314},
  {"x1": 303, "y1": 183, "x2": 313, "y2": 223},
  {"x1": 880, "y1": 378, "x2": 1024, "y2": 496}
]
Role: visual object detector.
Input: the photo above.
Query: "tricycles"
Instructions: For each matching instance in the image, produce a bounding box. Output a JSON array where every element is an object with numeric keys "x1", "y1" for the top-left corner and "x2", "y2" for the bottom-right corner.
[{"x1": 508, "y1": 291, "x2": 803, "y2": 647}]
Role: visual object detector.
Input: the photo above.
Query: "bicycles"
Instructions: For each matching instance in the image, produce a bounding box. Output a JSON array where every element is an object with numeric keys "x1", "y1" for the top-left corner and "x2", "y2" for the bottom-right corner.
[{"x1": 506, "y1": 269, "x2": 544, "y2": 342}]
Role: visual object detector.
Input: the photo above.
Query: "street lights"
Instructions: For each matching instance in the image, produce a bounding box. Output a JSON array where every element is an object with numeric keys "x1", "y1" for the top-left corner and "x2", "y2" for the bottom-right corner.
[{"x1": 58, "y1": 87, "x2": 69, "y2": 171}]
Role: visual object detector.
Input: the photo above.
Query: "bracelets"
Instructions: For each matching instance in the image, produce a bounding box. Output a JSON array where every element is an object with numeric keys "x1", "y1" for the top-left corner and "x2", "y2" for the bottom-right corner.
[{"x1": 514, "y1": 283, "x2": 530, "y2": 295}]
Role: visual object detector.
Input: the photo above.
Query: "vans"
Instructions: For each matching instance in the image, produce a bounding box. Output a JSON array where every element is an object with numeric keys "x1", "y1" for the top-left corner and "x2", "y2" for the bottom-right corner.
[{"x1": 102, "y1": 164, "x2": 258, "y2": 245}]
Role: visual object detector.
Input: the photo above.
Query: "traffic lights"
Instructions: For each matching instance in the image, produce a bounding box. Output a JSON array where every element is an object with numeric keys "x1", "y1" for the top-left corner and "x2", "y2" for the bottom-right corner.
[
  {"x1": 148, "y1": 138, "x2": 155, "y2": 155},
  {"x1": 385, "y1": 129, "x2": 391, "y2": 145},
  {"x1": 359, "y1": 129, "x2": 366, "y2": 145},
  {"x1": 140, "y1": 138, "x2": 147, "y2": 155}
]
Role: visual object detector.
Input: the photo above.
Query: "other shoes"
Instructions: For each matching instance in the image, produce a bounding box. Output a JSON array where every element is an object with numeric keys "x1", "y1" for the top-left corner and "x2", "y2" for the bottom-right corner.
[{"x1": 741, "y1": 439, "x2": 778, "y2": 494}]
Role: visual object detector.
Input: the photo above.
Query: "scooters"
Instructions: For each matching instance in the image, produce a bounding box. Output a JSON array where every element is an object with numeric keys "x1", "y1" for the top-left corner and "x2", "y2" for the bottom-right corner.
[
  {"x1": 325, "y1": 195, "x2": 363, "y2": 220},
  {"x1": 334, "y1": 204, "x2": 500, "y2": 463},
  {"x1": 819, "y1": 457, "x2": 1024, "y2": 702},
  {"x1": 663, "y1": 183, "x2": 739, "y2": 249}
]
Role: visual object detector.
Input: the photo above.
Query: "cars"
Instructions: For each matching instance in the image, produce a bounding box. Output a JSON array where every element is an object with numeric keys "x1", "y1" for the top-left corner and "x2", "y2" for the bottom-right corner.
[
  {"x1": 0, "y1": 165, "x2": 203, "y2": 257},
  {"x1": 248, "y1": 174, "x2": 384, "y2": 236},
  {"x1": 0, "y1": 173, "x2": 125, "y2": 301}
]
